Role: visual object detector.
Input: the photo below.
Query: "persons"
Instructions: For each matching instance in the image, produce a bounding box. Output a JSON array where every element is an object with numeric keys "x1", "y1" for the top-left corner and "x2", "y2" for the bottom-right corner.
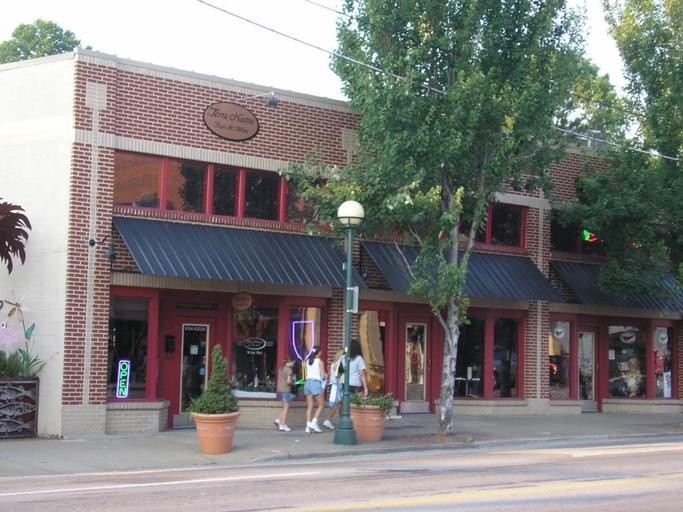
[
  {"x1": 273, "y1": 354, "x2": 298, "y2": 433},
  {"x1": 333, "y1": 338, "x2": 370, "y2": 403},
  {"x1": 321, "y1": 347, "x2": 348, "y2": 431},
  {"x1": 302, "y1": 345, "x2": 330, "y2": 435}
]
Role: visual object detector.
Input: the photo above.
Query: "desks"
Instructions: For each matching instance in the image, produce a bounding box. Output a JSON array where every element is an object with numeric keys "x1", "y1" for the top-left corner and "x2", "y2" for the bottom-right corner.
[{"x1": 454, "y1": 377, "x2": 480, "y2": 398}]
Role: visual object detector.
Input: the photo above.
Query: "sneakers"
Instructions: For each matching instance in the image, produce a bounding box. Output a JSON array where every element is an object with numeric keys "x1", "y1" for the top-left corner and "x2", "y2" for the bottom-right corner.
[
  {"x1": 323, "y1": 421, "x2": 335, "y2": 430},
  {"x1": 279, "y1": 425, "x2": 291, "y2": 432},
  {"x1": 305, "y1": 427, "x2": 312, "y2": 433},
  {"x1": 310, "y1": 422, "x2": 322, "y2": 432},
  {"x1": 274, "y1": 420, "x2": 280, "y2": 427}
]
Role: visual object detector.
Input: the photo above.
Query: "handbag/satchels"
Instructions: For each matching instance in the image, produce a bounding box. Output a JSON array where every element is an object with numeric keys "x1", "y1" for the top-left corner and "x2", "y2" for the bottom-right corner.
[{"x1": 340, "y1": 373, "x2": 345, "y2": 384}]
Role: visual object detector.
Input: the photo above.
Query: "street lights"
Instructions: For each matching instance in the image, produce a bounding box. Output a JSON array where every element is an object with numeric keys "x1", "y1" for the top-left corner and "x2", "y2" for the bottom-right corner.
[{"x1": 332, "y1": 200, "x2": 365, "y2": 445}]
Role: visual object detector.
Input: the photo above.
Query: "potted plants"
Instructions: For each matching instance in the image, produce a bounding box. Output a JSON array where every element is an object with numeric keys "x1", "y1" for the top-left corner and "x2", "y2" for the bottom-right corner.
[
  {"x1": 188, "y1": 345, "x2": 239, "y2": 455},
  {"x1": 349, "y1": 388, "x2": 392, "y2": 445}
]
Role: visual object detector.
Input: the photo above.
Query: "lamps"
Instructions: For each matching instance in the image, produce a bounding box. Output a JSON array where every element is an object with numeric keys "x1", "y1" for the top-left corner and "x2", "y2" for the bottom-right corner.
[
  {"x1": 88, "y1": 235, "x2": 117, "y2": 261},
  {"x1": 235, "y1": 92, "x2": 278, "y2": 109}
]
